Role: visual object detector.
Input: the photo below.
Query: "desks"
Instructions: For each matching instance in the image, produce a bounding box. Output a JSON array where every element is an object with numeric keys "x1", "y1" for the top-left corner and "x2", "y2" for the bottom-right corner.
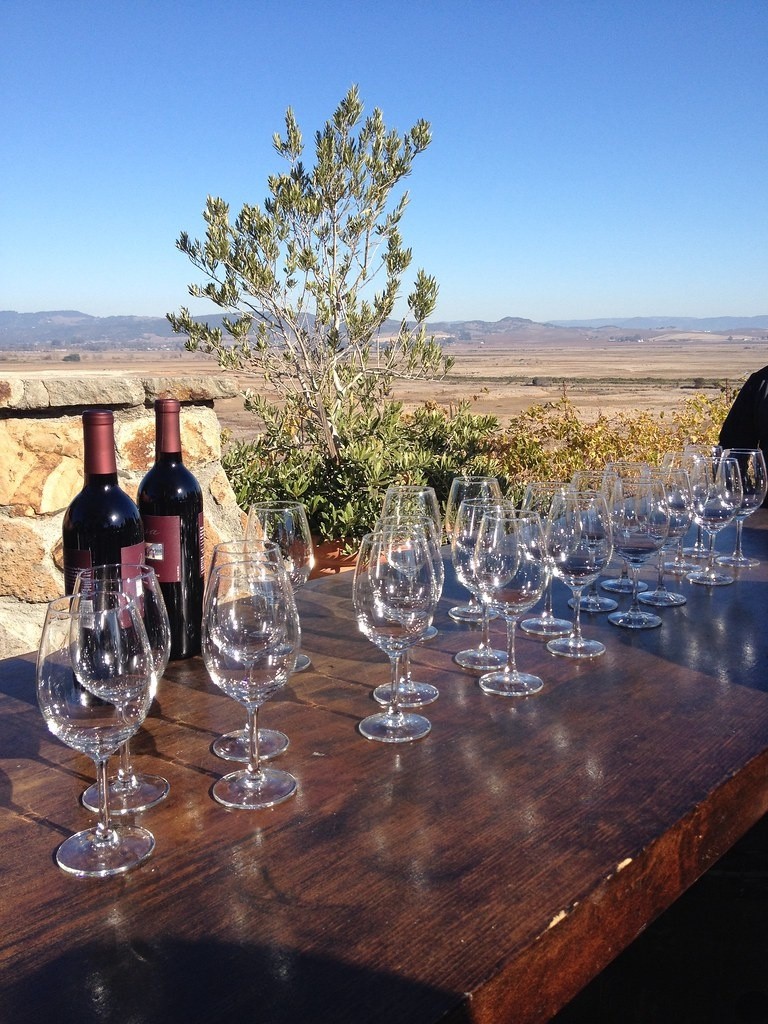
[{"x1": 0, "y1": 477, "x2": 768, "y2": 1023}]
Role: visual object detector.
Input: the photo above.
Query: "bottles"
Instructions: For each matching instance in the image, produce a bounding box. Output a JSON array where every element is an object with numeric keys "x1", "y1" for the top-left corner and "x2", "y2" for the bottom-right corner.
[
  {"x1": 64, "y1": 410, "x2": 149, "y2": 682},
  {"x1": 134, "y1": 399, "x2": 206, "y2": 662}
]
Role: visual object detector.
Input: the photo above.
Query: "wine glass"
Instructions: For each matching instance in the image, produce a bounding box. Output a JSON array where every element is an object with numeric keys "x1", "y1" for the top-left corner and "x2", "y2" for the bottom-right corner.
[
  {"x1": 350, "y1": 485, "x2": 446, "y2": 744},
  {"x1": 445, "y1": 476, "x2": 550, "y2": 699},
  {"x1": 519, "y1": 444, "x2": 768, "y2": 659},
  {"x1": 203, "y1": 499, "x2": 316, "y2": 812},
  {"x1": 36, "y1": 561, "x2": 172, "y2": 879}
]
